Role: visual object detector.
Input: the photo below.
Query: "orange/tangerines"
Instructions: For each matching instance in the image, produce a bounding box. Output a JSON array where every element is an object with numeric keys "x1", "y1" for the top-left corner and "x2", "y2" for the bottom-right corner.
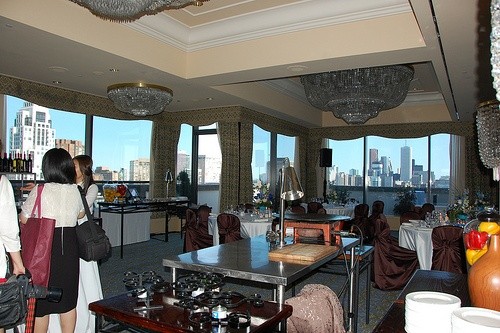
[{"x1": 104, "y1": 189, "x2": 117, "y2": 202}]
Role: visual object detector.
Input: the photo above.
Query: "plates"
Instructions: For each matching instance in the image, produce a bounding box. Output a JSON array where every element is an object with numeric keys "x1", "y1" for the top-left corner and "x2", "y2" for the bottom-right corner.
[{"x1": 404, "y1": 291, "x2": 500, "y2": 333}]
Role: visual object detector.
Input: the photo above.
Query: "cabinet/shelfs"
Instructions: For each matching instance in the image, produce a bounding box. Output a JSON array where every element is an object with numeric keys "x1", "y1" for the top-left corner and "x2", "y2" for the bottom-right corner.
[{"x1": 0, "y1": 172, "x2": 36, "y2": 206}]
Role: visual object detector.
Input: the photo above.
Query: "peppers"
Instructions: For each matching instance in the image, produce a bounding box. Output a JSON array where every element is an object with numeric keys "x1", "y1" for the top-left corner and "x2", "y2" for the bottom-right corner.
[{"x1": 465, "y1": 222, "x2": 500, "y2": 266}]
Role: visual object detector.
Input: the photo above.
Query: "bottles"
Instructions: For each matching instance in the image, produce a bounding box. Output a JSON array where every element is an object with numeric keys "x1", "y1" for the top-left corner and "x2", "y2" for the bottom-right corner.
[
  {"x1": 467, "y1": 234, "x2": 500, "y2": 310},
  {"x1": 116, "y1": 181, "x2": 127, "y2": 203},
  {"x1": 466, "y1": 205, "x2": 500, "y2": 266},
  {"x1": 103, "y1": 181, "x2": 116, "y2": 203},
  {"x1": 0, "y1": 151, "x2": 33, "y2": 173}
]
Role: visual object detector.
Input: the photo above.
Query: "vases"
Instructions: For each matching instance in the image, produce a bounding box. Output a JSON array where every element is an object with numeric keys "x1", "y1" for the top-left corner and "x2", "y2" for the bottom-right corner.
[{"x1": 259, "y1": 206, "x2": 266, "y2": 213}]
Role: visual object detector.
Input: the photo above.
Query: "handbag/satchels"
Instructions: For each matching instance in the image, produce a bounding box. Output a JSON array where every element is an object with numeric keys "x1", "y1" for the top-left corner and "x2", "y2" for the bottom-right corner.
[
  {"x1": 9, "y1": 182, "x2": 56, "y2": 289},
  {"x1": 0, "y1": 281, "x2": 27, "y2": 329},
  {"x1": 74, "y1": 185, "x2": 111, "y2": 262}
]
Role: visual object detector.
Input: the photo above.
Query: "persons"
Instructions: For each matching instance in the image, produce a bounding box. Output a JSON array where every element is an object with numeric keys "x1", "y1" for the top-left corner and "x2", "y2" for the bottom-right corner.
[{"x1": 0, "y1": 148, "x2": 104, "y2": 333}]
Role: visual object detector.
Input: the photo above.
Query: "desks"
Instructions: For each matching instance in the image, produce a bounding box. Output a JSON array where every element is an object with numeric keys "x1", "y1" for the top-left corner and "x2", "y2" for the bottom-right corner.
[
  {"x1": 370, "y1": 270, "x2": 471, "y2": 333},
  {"x1": 322, "y1": 203, "x2": 370, "y2": 219},
  {"x1": 398, "y1": 223, "x2": 438, "y2": 270},
  {"x1": 97, "y1": 201, "x2": 193, "y2": 259},
  {"x1": 163, "y1": 235, "x2": 360, "y2": 308},
  {"x1": 88, "y1": 282, "x2": 293, "y2": 333},
  {"x1": 208, "y1": 213, "x2": 273, "y2": 246},
  {"x1": 336, "y1": 245, "x2": 376, "y2": 333},
  {"x1": 272, "y1": 214, "x2": 351, "y2": 247}
]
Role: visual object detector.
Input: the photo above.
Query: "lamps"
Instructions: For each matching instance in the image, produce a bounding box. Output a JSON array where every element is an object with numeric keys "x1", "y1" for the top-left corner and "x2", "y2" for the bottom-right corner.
[
  {"x1": 299, "y1": 65, "x2": 415, "y2": 125},
  {"x1": 163, "y1": 168, "x2": 174, "y2": 199},
  {"x1": 490, "y1": 0, "x2": 500, "y2": 101},
  {"x1": 277, "y1": 157, "x2": 304, "y2": 250},
  {"x1": 474, "y1": 100, "x2": 500, "y2": 182},
  {"x1": 319, "y1": 148, "x2": 332, "y2": 204},
  {"x1": 107, "y1": 82, "x2": 173, "y2": 117},
  {"x1": 68, "y1": 0, "x2": 210, "y2": 23}
]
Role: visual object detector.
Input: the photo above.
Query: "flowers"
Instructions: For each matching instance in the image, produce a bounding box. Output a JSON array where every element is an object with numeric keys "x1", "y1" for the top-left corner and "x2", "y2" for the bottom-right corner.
[
  {"x1": 326, "y1": 188, "x2": 352, "y2": 203},
  {"x1": 444, "y1": 199, "x2": 479, "y2": 224},
  {"x1": 464, "y1": 222, "x2": 500, "y2": 266},
  {"x1": 252, "y1": 179, "x2": 270, "y2": 206}
]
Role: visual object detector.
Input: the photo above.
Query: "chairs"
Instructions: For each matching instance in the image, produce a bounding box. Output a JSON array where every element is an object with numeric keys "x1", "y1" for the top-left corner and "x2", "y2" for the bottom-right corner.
[{"x1": 182, "y1": 201, "x2": 479, "y2": 290}]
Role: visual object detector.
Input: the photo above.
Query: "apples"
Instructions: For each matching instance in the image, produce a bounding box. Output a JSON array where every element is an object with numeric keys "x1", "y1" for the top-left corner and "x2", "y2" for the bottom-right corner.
[{"x1": 116, "y1": 186, "x2": 126, "y2": 197}]
